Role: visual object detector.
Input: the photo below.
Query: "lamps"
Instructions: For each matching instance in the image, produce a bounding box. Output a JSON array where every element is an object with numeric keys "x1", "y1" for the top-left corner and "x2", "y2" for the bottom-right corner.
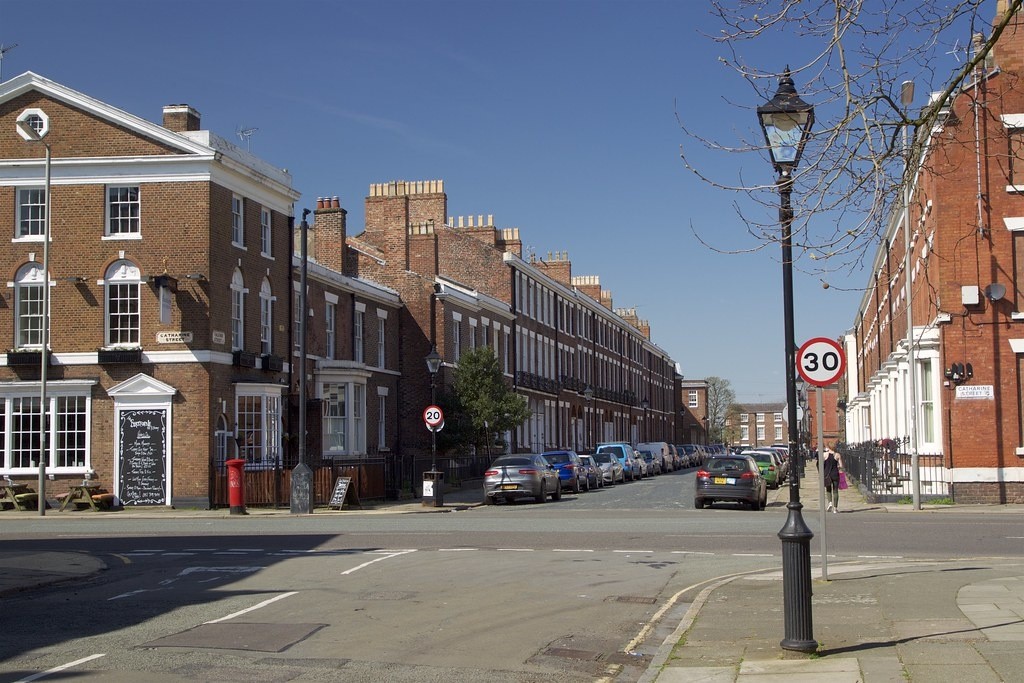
[
  {"x1": 188, "y1": 274, "x2": 207, "y2": 279},
  {"x1": 66, "y1": 277, "x2": 89, "y2": 284}
]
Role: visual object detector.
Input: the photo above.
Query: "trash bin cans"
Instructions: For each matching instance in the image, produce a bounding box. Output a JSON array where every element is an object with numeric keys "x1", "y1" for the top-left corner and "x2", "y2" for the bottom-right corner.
[
  {"x1": 422, "y1": 471, "x2": 444, "y2": 507},
  {"x1": 799, "y1": 456, "x2": 805, "y2": 478}
]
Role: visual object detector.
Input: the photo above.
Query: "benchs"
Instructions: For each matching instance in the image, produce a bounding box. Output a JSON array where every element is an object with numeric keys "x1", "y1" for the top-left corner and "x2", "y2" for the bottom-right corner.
[
  {"x1": 15, "y1": 492, "x2": 38, "y2": 498},
  {"x1": 55, "y1": 492, "x2": 76, "y2": 501},
  {"x1": 92, "y1": 493, "x2": 114, "y2": 500}
]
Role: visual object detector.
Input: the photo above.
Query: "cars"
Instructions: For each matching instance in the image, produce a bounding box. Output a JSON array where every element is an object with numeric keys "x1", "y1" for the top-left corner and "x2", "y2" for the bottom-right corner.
[
  {"x1": 591, "y1": 453, "x2": 626, "y2": 486},
  {"x1": 640, "y1": 450, "x2": 662, "y2": 477},
  {"x1": 483, "y1": 453, "x2": 562, "y2": 505},
  {"x1": 575, "y1": 455, "x2": 605, "y2": 489},
  {"x1": 694, "y1": 454, "x2": 769, "y2": 511},
  {"x1": 634, "y1": 450, "x2": 649, "y2": 478},
  {"x1": 539, "y1": 450, "x2": 589, "y2": 494},
  {"x1": 666, "y1": 440, "x2": 810, "y2": 490}
]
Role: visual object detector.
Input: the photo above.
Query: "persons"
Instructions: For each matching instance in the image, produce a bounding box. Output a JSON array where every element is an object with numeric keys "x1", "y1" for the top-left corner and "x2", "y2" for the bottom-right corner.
[{"x1": 812, "y1": 445, "x2": 843, "y2": 513}]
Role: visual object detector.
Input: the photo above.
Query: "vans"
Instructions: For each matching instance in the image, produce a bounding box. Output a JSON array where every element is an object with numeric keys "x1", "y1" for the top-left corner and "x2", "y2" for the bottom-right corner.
[{"x1": 595, "y1": 443, "x2": 643, "y2": 483}]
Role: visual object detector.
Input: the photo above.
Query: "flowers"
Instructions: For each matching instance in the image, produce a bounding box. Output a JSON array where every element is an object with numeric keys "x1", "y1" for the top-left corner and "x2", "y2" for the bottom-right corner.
[
  {"x1": 99, "y1": 345, "x2": 143, "y2": 350},
  {"x1": 3, "y1": 348, "x2": 53, "y2": 353}
]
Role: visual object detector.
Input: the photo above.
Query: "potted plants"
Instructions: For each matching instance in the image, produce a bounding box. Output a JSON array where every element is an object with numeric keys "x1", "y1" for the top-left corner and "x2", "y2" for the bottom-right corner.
[
  {"x1": 262, "y1": 354, "x2": 284, "y2": 371},
  {"x1": 233, "y1": 350, "x2": 256, "y2": 368}
]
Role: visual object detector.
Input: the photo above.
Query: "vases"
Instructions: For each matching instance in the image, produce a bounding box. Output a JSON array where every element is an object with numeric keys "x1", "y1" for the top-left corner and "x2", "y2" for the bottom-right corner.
[
  {"x1": 97, "y1": 350, "x2": 141, "y2": 363},
  {"x1": 6, "y1": 352, "x2": 51, "y2": 366}
]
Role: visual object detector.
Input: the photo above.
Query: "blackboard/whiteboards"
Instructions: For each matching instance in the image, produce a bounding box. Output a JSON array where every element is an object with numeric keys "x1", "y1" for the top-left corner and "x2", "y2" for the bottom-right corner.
[{"x1": 328, "y1": 477, "x2": 361, "y2": 507}]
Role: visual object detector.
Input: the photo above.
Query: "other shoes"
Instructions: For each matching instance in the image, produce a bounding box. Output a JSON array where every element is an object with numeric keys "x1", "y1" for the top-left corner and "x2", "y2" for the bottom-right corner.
[
  {"x1": 833, "y1": 510, "x2": 840, "y2": 513},
  {"x1": 826, "y1": 504, "x2": 832, "y2": 512}
]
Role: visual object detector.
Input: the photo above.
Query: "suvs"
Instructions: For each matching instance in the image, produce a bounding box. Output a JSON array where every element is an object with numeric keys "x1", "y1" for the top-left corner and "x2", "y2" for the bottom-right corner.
[{"x1": 634, "y1": 441, "x2": 674, "y2": 475}]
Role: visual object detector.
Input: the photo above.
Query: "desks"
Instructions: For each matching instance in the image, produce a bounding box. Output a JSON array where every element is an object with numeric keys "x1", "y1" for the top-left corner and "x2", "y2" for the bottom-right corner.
[
  {"x1": 0, "y1": 483, "x2": 32, "y2": 511},
  {"x1": 59, "y1": 483, "x2": 109, "y2": 512}
]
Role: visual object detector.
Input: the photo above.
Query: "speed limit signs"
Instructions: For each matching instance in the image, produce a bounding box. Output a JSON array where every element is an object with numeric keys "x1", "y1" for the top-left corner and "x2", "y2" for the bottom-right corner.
[
  {"x1": 796, "y1": 337, "x2": 846, "y2": 388},
  {"x1": 424, "y1": 404, "x2": 444, "y2": 426}
]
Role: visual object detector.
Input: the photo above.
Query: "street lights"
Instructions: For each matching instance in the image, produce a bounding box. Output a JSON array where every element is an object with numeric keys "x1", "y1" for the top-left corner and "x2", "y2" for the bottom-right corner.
[
  {"x1": 423, "y1": 345, "x2": 444, "y2": 471},
  {"x1": 731, "y1": 430, "x2": 736, "y2": 446},
  {"x1": 795, "y1": 373, "x2": 806, "y2": 478},
  {"x1": 702, "y1": 416, "x2": 708, "y2": 446},
  {"x1": 583, "y1": 384, "x2": 594, "y2": 454},
  {"x1": 642, "y1": 397, "x2": 649, "y2": 443},
  {"x1": 15, "y1": 120, "x2": 53, "y2": 516},
  {"x1": 678, "y1": 405, "x2": 687, "y2": 445},
  {"x1": 901, "y1": 80, "x2": 923, "y2": 509},
  {"x1": 754, "y1": 64, "x2": 819, "y2": 652}
]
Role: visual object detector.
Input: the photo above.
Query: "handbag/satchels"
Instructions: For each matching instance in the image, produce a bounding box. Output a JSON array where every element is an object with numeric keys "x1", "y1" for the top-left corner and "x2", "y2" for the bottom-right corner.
[
  {"x1": 838, "y1": 470, "x2": 848, "y2": 490},
  {"x1": 823, "y1": 475, "x2": 831, "y2": 487}
]
